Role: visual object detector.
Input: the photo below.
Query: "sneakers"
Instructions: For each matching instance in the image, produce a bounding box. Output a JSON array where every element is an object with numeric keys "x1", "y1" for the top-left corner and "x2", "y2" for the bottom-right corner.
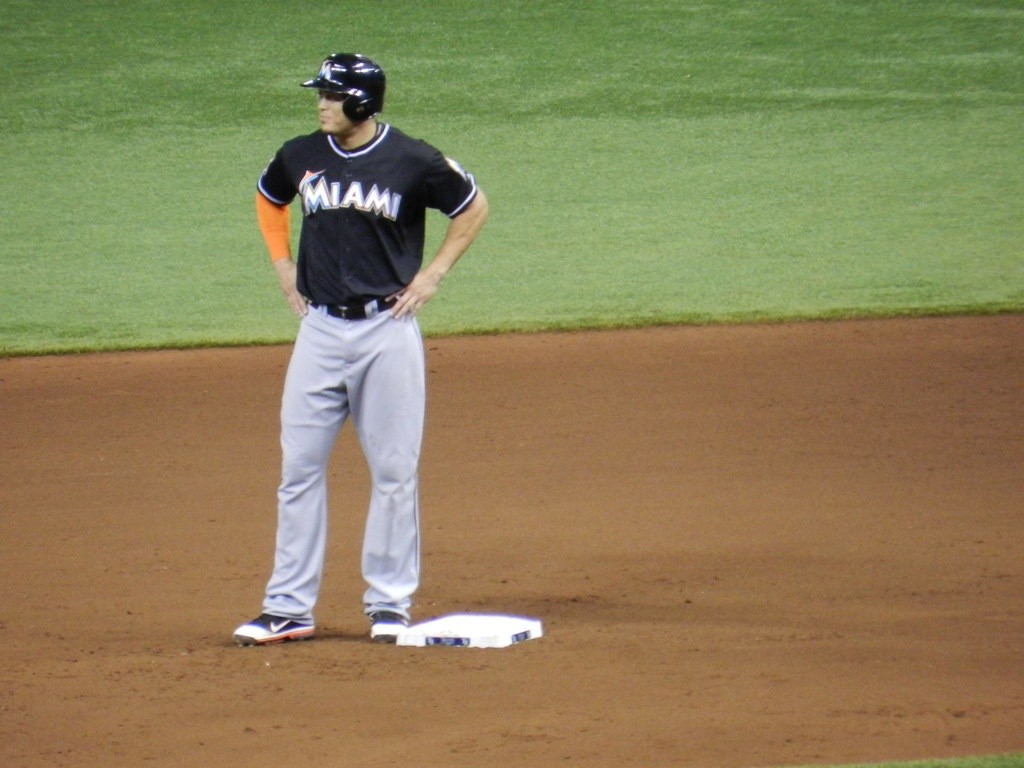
[
  {"x1": 232, "y1": 611, "x2": 316, "y2": 642},
  {"x1": 370, "y1": 610, "x2": 410, "y2": 642}
]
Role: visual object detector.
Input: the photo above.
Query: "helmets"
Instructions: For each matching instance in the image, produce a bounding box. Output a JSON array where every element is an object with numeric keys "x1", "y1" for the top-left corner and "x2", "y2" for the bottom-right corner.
[{"x1": 299, "y1": 53, "x2": 385, "y2": 125}]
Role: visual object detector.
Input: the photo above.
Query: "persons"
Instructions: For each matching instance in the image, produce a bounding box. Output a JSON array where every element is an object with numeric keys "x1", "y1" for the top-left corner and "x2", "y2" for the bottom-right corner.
[{"x1": 232, "y1": 54, "x2": 488, "y2": 646}]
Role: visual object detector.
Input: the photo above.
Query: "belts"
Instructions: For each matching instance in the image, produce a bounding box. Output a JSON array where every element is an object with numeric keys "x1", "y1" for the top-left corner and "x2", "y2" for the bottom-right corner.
[{"x1": 305, "y1": 288, "x2": 406, "y2": 321}]
